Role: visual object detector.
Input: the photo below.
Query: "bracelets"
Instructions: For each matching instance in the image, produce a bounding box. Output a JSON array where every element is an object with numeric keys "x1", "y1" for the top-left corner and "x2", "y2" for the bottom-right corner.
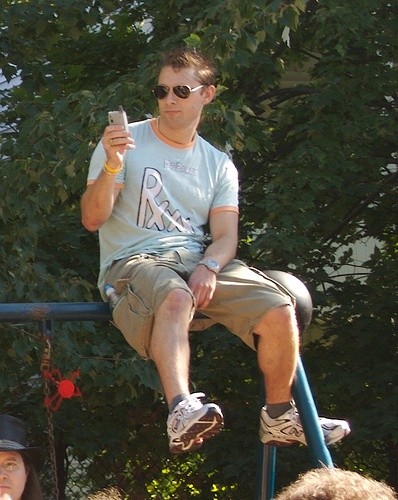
[{"x1": 101, "y1": 160, "x2": 124, "y2": 175}]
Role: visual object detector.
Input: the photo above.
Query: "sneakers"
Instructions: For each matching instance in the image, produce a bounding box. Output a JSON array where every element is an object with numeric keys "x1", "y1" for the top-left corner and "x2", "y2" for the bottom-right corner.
[
  {"x1": 258, "y1": 405, "x2": 350, "y2": 447},
  {"x1": 166, "y1": 392, "x2": 225, "y2": 454}
]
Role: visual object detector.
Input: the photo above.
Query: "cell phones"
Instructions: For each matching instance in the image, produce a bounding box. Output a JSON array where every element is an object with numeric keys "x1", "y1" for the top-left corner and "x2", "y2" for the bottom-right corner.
[{"x1": 107, "y1": 104, "x2": 128, "y2": 147}]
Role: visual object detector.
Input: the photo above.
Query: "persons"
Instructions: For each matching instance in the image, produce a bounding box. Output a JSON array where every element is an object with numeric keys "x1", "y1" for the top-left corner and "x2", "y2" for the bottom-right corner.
[
  {"x1": 0, "y1": 414, "x2": 47, "y2": 500},
  {"x1": 79, "y1": 48, "x2": 350, "y2": 453}
]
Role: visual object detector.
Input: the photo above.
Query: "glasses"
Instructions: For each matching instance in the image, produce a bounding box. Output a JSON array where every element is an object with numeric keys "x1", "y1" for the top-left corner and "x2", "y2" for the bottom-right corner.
[{"x1": 152, "y1": 85, "x2": 204, "y2": 99}]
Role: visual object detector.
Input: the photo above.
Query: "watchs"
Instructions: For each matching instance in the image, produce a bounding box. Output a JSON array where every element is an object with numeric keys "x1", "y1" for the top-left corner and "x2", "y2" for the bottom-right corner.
[{"x1": 197, "y1": 257, "x2": 221, "y2": 273}]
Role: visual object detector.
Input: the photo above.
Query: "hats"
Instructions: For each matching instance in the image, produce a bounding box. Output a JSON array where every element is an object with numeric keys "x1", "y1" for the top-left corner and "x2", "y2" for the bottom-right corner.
[{"x1": 0, "y1": 415, "x2": 40, "y2": 451}]
